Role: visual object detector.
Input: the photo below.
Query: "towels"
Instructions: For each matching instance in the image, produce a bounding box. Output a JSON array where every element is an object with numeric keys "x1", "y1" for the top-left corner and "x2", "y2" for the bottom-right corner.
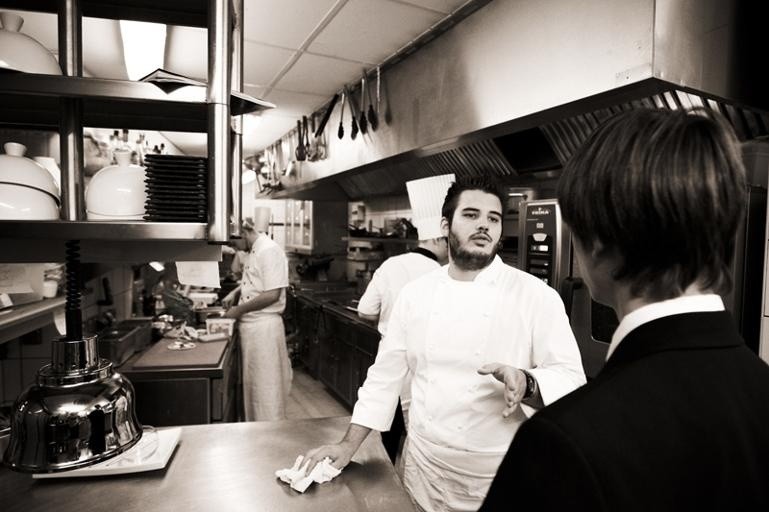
[{"x1": 276, "y1": 449, "x2": 361, "y2": 494}]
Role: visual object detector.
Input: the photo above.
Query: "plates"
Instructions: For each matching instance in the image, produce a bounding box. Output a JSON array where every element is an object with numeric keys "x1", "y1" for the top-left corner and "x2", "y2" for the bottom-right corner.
[
  {"x1": 33, "y1": 427, "x2": 182, "y2": 478},
  {"x1": 143, "y1": 154, "x2": 208, "y2": 222}
]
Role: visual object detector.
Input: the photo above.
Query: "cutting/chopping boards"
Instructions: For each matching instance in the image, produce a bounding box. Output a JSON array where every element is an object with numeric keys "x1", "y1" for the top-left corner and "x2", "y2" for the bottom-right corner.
[{"x1": 133, "y1": 336, "x2": 229, "y2": 370}]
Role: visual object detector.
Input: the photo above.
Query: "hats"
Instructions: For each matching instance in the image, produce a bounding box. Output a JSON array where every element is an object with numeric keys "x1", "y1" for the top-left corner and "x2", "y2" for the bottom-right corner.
[
  {"x1": 243, "y1": 206, "x2": 270, "y2": 232},
  {"x1": 407, "y1": 174, "x2": 457, "y2": 241}
]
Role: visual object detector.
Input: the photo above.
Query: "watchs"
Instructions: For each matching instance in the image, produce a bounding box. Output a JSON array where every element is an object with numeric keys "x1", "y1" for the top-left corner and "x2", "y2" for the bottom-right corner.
[{"x1": 521, "y1": 366, "x2": 536, "y2": 401}]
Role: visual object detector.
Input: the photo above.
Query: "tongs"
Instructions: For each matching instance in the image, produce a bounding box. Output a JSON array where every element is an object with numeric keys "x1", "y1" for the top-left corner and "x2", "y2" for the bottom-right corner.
[
  {"x1": 315, "y1": 92, "x2": 338, "y2": 136},
  {"x1": 300, "y1": 115, "x2": 309, "y2": 154},
  {"x1": 339, "y1": 83, "x2": 357, "y2": 139},
  {"x1": 359, "y1": 68, "x2": 374, "y2": 134}
]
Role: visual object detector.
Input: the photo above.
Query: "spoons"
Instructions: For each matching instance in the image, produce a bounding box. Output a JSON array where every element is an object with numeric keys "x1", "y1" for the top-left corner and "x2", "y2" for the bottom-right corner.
[
  {"x1": 263, "y1": 146, "x2": 271, "y2": 187},
  {"x1": 270, "y1": 142, "x2": 280, "y2": 188},
  {"x1": 295, "y1": 121, "x2": 303, "y2": 160},
  {"x1": 372, "y1": 66, "x2": 380, "y2": 132}
]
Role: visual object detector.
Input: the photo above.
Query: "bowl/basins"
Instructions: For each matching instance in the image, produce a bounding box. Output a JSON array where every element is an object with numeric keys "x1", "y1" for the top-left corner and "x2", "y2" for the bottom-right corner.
[
  {"x1": 0, "y1": 142, "x2": 60, "y2": 204},
  {"x1": 86, "y1": 150, "x2": 149, "y2": 215},
  {"x1": 0, "y1": 183, "x2": 59, "y2": 220},
  {"x1": 0, "y1": 11, "x2": 63, "y2": 75},
  {"x1": 87, "y1": 212, "x2": 147, "y2": 220}
]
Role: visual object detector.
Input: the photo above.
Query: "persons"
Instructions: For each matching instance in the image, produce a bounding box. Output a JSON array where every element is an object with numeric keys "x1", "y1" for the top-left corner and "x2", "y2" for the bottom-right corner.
[
  {"x1": 232, "y1": 206, "x2": 271, "y2": 276},
  {"x1": 478, "y1": 106, "x2": 768, "y2": 512},
  {"x1": 222, "y1": 216, "x2": 293, "y2": 421},
  {"x1": 358, "y1": 172, "x2": 451, "y2": 342},
  {"x1": 295, "y1": 172, "x2": 588, "y2": 511}
]
